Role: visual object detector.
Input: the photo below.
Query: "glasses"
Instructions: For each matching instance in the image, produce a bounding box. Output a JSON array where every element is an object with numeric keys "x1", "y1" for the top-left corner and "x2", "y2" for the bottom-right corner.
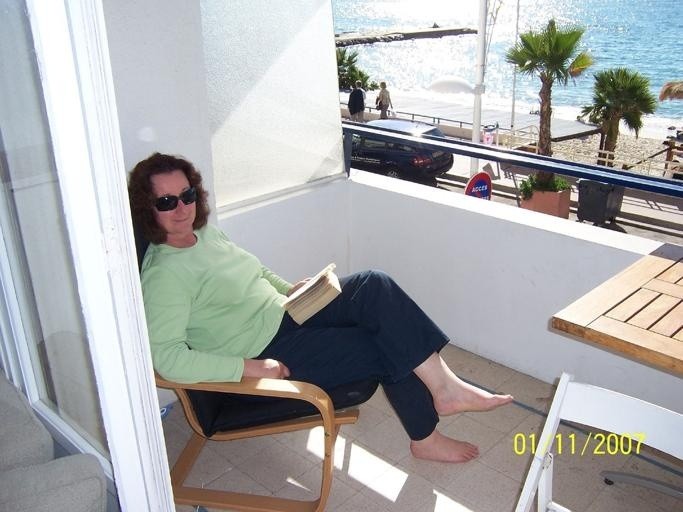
[{"x1": 156, "y1": 187, "x2": 198, "y2": 212}]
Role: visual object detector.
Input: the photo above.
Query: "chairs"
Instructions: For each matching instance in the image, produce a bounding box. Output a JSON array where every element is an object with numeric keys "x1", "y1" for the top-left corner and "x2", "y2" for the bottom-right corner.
[
  {"x1": 511, "y1": 368, "x2": 682, "y2": 511},
  {"x1": 143, "y1": 360, "x2": 359, "y2": 512}
]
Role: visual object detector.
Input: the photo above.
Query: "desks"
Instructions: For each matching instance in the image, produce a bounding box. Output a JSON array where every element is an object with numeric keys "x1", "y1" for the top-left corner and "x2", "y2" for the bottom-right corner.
[{"x1": 544, "y1": 242, "x2": 682, "y2": 381}]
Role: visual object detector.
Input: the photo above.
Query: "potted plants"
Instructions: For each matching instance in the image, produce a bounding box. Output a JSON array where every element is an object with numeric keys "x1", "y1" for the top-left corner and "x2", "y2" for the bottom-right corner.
[
  {"x1": 504, "y1": 16, "x2": 595, "y2": 220},
  {"x1": 576, "y1": 67, "x2": 659, "y2": 223}
]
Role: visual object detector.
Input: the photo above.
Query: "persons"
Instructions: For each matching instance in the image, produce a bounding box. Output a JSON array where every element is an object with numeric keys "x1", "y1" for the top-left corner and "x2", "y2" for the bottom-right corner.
[
  {"x1": 376, "y1": 81, "x2": 392, "y2": 119},
  {"x1": 350, "y1": 80, "x2": 367, "y2": 122},
  {"x1": 127, "y1": 150, "x2": 514, "y2": 462}
]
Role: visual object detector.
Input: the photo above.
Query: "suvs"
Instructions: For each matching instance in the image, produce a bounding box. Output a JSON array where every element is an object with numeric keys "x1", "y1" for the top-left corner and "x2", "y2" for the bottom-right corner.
[{"x1": 343, "y1": 117, "x2": 454, "y2": 180}]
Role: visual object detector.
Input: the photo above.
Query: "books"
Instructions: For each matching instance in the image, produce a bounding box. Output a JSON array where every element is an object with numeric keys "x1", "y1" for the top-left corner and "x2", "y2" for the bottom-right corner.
[{"x1": 280, "y1": 262, "x2": 343, "y2": 326}]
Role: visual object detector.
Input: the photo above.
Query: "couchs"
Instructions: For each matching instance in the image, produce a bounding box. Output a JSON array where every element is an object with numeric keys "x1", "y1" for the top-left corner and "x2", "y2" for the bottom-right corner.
[{"x1": 0, "y1": 369, "x2": 112, "y2": 512}]
